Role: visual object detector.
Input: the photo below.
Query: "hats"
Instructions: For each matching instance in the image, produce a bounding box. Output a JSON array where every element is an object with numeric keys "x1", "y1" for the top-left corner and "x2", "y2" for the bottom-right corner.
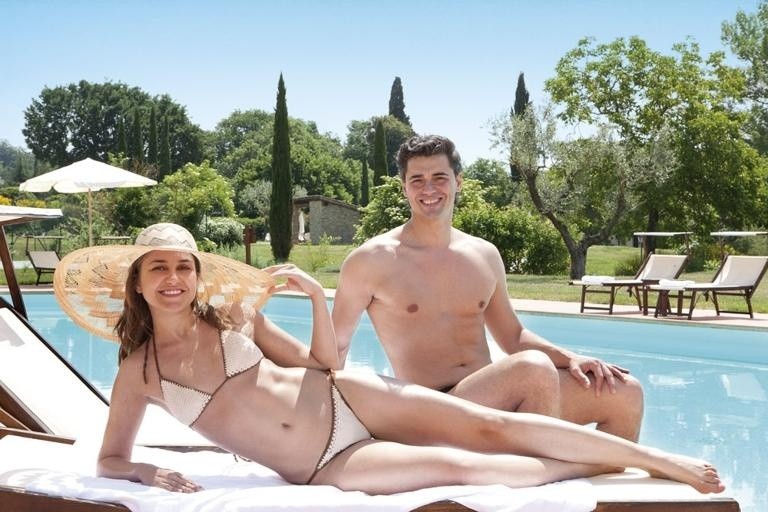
[{"x1": 51, "y1": 222, "x2": 278, "y2": 343}]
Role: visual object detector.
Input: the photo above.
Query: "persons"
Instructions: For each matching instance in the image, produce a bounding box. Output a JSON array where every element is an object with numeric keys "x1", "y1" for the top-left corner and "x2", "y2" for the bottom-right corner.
[
  {"x1": 329, "y1": 135, "x2": 643, "y2": 443},
  {"x1": 97, "y1": 223, "x2": 726, "y2": 494}
]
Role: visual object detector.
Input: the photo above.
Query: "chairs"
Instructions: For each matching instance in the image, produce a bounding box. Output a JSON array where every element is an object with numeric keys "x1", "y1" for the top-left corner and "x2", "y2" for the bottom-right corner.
[
  {"x1": 0, "y1": 427, "x2": 741, "y2": 512},
  {"x1": 0, "y1": 296, "x2": 219, "y2": 447},
  {"x1": 25, "y1": 249, "x2": 100, "y2": 286},
  {"x1": 568, "y1": 251, "x2": 768, "y2": 320}
]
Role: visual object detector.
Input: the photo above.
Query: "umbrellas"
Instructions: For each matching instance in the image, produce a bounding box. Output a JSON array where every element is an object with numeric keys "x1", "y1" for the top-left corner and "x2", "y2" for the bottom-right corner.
[{"x1": 19, "y1": 158, "x2": 158, "y2": 248}]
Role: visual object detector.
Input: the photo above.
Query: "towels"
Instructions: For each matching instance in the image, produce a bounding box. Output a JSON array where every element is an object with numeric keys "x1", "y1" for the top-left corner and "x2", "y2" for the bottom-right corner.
[{"x1": 26, "y1": 435, "x2": 597, "y2": 512}]
